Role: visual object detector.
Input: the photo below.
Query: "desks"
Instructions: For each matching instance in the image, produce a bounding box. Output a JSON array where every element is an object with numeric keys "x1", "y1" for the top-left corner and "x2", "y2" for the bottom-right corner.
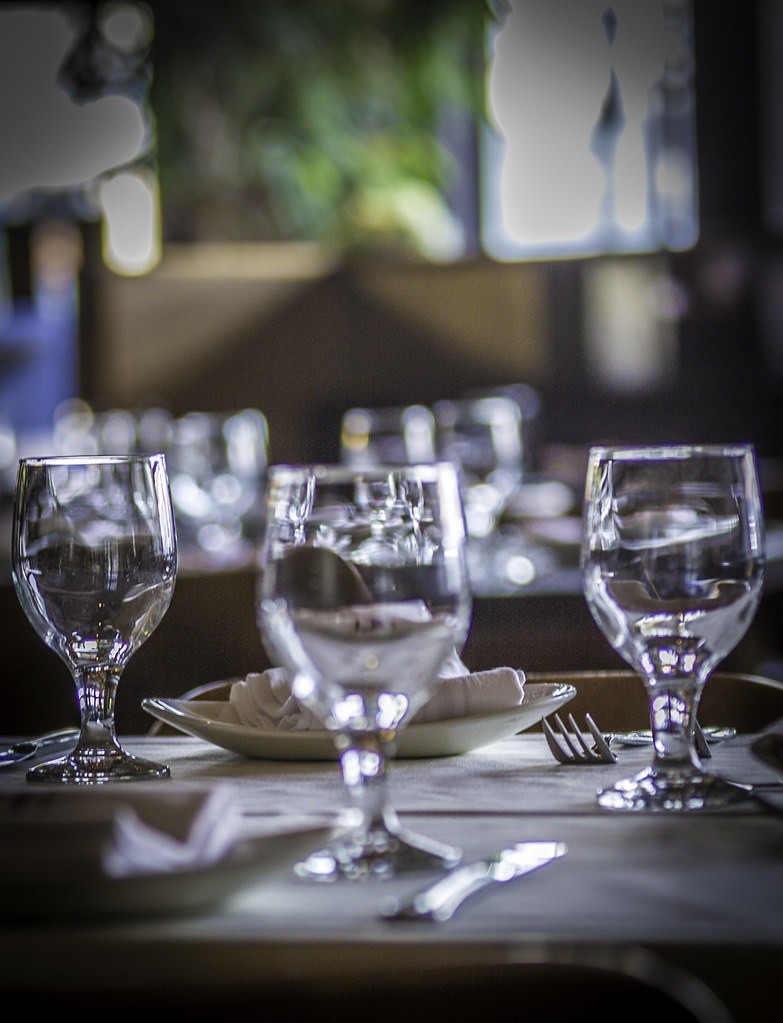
[
  {"x1": 0, "y1": 702, "x2": 782, "y2": 1022},
  {"x1": 1, "y1": 507, "x2": 782, "y2": 742}
]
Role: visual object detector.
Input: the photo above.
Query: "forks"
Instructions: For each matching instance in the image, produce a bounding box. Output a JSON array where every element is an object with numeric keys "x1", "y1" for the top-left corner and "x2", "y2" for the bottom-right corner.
[{"x1": 541, "y1": 713, "x2": 736, "y2": 764}]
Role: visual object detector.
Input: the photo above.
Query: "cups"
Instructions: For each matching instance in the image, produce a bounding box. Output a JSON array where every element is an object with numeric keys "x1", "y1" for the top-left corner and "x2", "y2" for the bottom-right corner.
[{"x1": 342, "y1": 407, "x2": 435, "y2": 471}]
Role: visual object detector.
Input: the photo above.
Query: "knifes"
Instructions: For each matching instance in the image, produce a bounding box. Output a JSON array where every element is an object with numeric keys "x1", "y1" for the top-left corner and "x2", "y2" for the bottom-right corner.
[{"x1": 407, "y1": 839, "x2": 569, "y2": 924}]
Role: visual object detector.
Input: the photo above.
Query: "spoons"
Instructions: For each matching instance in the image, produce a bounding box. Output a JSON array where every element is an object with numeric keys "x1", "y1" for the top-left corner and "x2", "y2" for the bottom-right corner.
[{"x1": 0, "y1": 728, "x2": 80, "y2": 768}]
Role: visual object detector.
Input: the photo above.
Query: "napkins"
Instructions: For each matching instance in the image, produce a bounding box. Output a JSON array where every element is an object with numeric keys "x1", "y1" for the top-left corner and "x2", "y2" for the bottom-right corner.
[{"x1": 227, "y1": 640, "x2": 527, "y2": 732}]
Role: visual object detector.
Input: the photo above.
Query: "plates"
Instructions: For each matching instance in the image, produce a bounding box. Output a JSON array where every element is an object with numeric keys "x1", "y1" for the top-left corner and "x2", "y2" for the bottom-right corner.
[
  {"x1": 0, "y1": 784, "x2": 362, "y2": 924},
  {"x1": 526, "y1": 517, "x2": 737, "y2": 549},
  {"x1": 141, "y1": 683, "x2": 576, "y2": 758}
]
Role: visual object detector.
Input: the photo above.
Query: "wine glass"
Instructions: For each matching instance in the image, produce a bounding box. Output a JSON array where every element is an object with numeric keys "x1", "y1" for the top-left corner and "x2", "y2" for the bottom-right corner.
[
  {"x1": 584, "y1": 444, "x2": 766, "y2": 808},
  {"x1": 175, "y1": 415, "x2": 273, "y2": 573},
  {"x1": 258, "y1": 464, "x2": 470, "y2": 885},
  {"x1": 440, "y1": 399, "x2": 528, "y2": 588},
  {"x1": 11, "y1": 454, "x2": 176, "y2": 782}
]
